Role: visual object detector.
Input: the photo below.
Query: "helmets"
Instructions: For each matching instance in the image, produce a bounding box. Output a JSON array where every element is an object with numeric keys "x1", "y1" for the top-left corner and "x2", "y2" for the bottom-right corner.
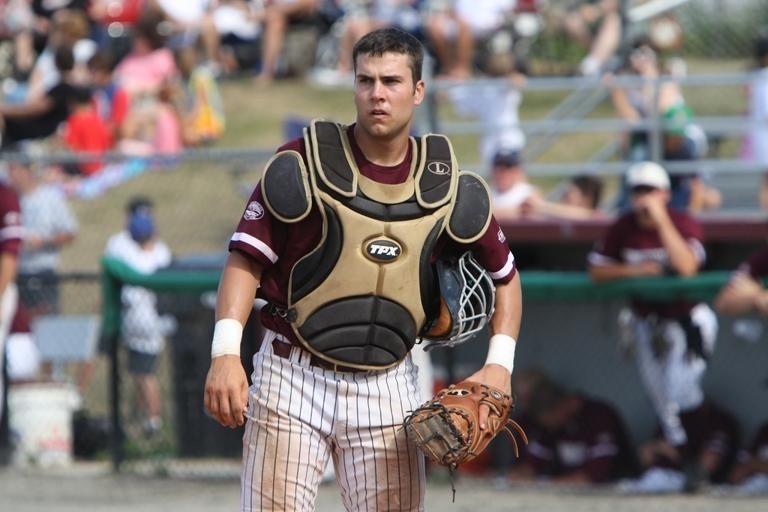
[{"x1": 424, "y1": 251, "x2": 496, "y2": 352}]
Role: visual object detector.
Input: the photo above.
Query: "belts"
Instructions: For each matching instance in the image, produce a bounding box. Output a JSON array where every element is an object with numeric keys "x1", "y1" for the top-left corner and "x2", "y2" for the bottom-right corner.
[{"x1": 272, "y1": 337, "x2": 372, "y2": 374}]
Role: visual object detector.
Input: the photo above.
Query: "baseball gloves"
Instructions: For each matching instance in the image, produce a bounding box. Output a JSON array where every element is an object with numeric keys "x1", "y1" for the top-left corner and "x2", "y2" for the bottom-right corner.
[{"x1": 402, "y1": 381, "x2": 529, "y2": 503}]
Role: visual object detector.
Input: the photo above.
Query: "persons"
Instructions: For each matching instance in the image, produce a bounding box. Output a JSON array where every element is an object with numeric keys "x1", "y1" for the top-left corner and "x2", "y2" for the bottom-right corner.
[
  {"x1": 205, "y1": 28, "x2": 521, "y2": 512},
  {"x1": 0, "y1": 0, "x2": 768, "y2": 490}
]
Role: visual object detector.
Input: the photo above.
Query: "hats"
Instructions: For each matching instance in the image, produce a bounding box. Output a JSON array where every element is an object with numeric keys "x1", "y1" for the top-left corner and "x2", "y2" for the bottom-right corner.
[
  {"x1": 130, "y1": 216, "x2": 151, "y2": 240},
  {"x1": 626, "y1": 161, "x2": 670, "y2": 190},
  {"x1": 495, "y1": 150, "x2": 518, "y2": 165}
]
